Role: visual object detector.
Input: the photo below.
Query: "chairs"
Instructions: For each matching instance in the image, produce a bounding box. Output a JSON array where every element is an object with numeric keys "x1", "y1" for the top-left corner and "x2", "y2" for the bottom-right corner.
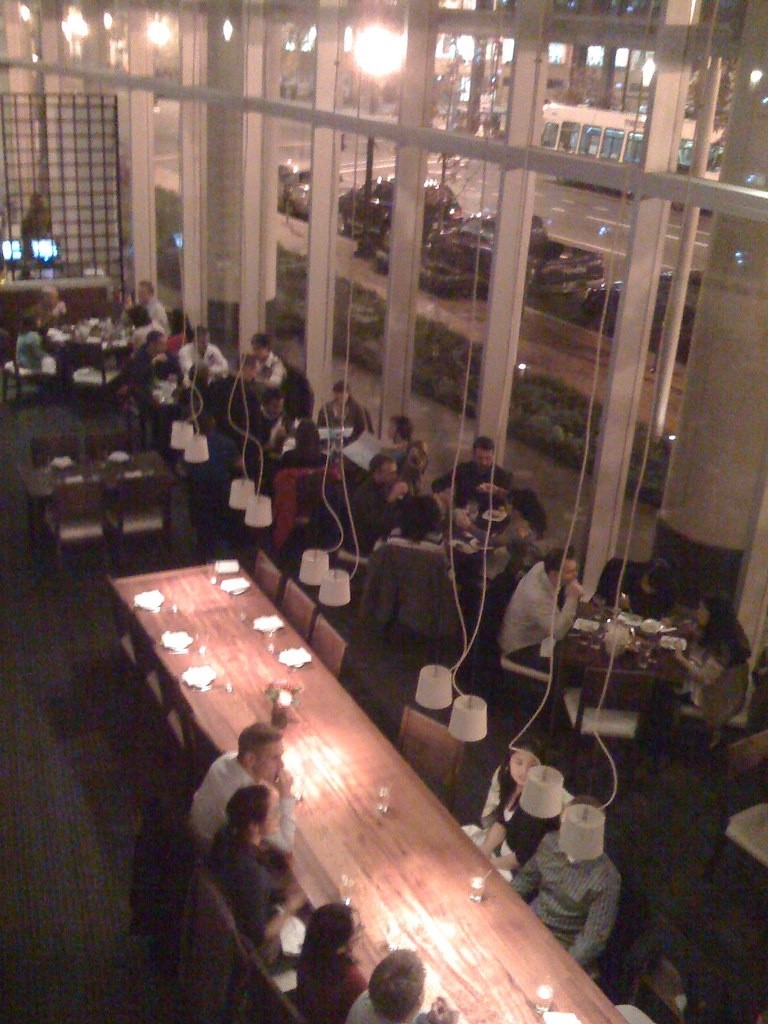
[{"x1": 1, "y1": 329, "x2": 768, "y2": 1024}]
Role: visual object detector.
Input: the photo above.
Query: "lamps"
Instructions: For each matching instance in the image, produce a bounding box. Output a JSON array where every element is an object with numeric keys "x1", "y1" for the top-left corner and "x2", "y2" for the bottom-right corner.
[{"x1": 170, "y1": 1, "x2": 717, "y2": 861}]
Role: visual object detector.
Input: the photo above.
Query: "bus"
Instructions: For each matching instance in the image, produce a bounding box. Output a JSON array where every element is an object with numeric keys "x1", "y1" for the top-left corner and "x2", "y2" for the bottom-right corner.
[{"x1": 535, "y1": 104, "x2": 724, "y2": 218}]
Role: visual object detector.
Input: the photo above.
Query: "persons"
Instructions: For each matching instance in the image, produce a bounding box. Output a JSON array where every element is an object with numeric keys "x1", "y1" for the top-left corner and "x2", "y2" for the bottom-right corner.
[
  {"x1": 460, "y1": 741, "x2": 620, "y2": 974},
  {"x1": 500, "y1": 545, "x2": 750, "y2": 762},
  {"x1": 16, "y1": 280, "x2": 548, "y2": 658},
  {"x1": 190, "y1": 724, "x2": 458, "y2": 1024},
  {"x1": 20, "y1": 193, "x2": 51, "y2": 259}
]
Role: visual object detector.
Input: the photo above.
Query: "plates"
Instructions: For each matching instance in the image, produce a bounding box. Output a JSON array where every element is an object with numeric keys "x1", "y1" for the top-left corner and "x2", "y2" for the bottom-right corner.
[
  {"x1": 573, "y1": 617, "x2": 599, "y2": 633},
  {"x1": 660, "y1": 634, "x2": 687, "y2": 652},
  {"x1": 617, "y1": 611, "x2": 642, "y2": 627},
  {"x1": 483, "y1": 509, "x2": 508, "y2": 523}
]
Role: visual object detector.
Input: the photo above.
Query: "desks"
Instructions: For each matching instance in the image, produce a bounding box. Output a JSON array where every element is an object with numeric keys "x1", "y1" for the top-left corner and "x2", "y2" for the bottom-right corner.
[
  {"x1": 49, "y1": 321, "x2": 133, "y2": 403},
  {"x1": 112, "y1": 562, "x2": 628, "y2": 1023},
  {"x1": 554, "y1": 602, "x2": 686, "y2": 717},
  {"x1": 23, "y1": 448, "x2": 170, "y2": 564}
]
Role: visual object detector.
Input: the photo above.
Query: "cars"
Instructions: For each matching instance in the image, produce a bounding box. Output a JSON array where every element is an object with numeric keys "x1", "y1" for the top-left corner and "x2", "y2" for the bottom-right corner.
[
  {"x1": 276, "y1": 161, "x2": 316, "y2": 220},
  {"x1": 335, "y1": 175, "x2": 703, "y2": 371}
]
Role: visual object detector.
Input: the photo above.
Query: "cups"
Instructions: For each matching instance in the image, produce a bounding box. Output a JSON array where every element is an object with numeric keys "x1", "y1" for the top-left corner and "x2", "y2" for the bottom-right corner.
[
  {"x1": 469, "y1": 876, "x2": 485, "y2": 903},
  {"x1": 195, "y1": 630, "x2": 213, "y2": 664},
  {"x1": 261, "y1": 628, "x2": 279, "y2": 654},
  {"x1": 375, "y1": 786, "x2": 390, "y2": 814},
  {"x1": 534, "y1": 985, "x2": 554, "y2": 1015}
]
